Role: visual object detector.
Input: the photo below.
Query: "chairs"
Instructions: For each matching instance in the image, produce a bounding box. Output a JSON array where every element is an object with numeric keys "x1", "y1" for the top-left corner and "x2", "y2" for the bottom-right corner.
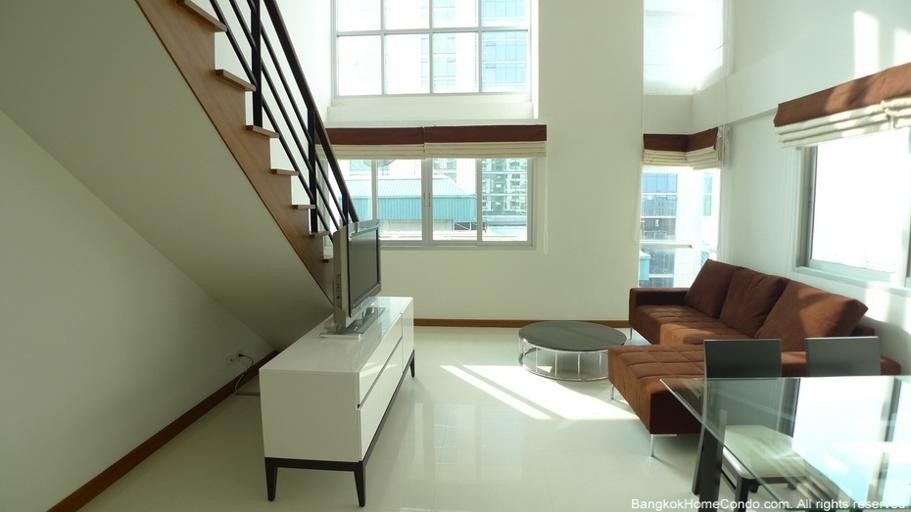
[
  {"x1": 689, "y1": 338, "x2": 809, "y2": 511},
  {"x1": 800, "y1": 335, "x2": 883, "y2": 510}
]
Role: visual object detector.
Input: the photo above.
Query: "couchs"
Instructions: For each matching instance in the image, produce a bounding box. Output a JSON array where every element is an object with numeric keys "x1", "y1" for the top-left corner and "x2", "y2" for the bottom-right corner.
[{"x1": 606, "y1": 257, "x2": 902, "y2": 456}]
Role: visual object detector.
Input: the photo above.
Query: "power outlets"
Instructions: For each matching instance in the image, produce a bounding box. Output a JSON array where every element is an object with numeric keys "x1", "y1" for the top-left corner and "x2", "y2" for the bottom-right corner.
[{"x1": 235, "y1": 350, "x2": 247, "y2": 362}]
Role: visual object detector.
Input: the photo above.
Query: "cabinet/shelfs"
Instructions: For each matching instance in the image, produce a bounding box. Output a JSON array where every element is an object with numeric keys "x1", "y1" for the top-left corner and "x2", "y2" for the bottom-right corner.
[{"x1": 259, "y1": 296, "x2": 418, "y2": 508}]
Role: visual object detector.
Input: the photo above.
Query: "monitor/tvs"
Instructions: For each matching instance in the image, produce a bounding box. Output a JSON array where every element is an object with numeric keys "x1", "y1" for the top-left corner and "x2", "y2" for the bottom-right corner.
[{"x1": 332, "y1": 217, "x2": 385, "y2": 326}]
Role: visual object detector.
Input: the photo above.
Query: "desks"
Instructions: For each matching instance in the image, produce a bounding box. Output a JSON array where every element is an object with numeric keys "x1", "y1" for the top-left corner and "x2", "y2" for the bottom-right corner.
[{"x1": 654, "y1": 375, "x2": 911, "y2": 511}]
